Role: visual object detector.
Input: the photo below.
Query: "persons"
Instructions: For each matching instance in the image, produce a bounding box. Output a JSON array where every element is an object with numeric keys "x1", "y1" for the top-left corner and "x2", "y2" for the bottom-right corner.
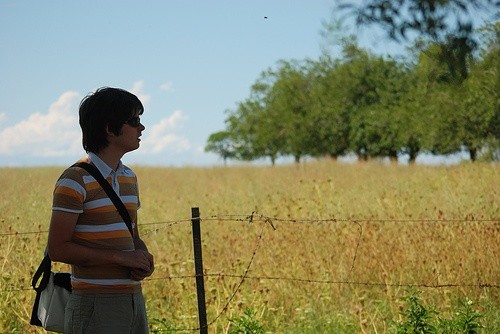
[{"x1": 29, "y1": 85, "x2": 155, "y2": 334}]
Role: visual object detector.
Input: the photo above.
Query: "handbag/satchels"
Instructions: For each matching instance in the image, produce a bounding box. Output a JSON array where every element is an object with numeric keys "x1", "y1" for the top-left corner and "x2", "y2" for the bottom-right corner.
[{"x1": 29, "y1": 271, "x2": 71, "y2": 334}]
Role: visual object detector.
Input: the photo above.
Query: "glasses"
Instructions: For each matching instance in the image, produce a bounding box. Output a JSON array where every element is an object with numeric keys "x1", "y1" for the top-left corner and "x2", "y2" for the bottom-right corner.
[{"x1": 118, "y1": 115, "x2": 141, "y2": 128}]
